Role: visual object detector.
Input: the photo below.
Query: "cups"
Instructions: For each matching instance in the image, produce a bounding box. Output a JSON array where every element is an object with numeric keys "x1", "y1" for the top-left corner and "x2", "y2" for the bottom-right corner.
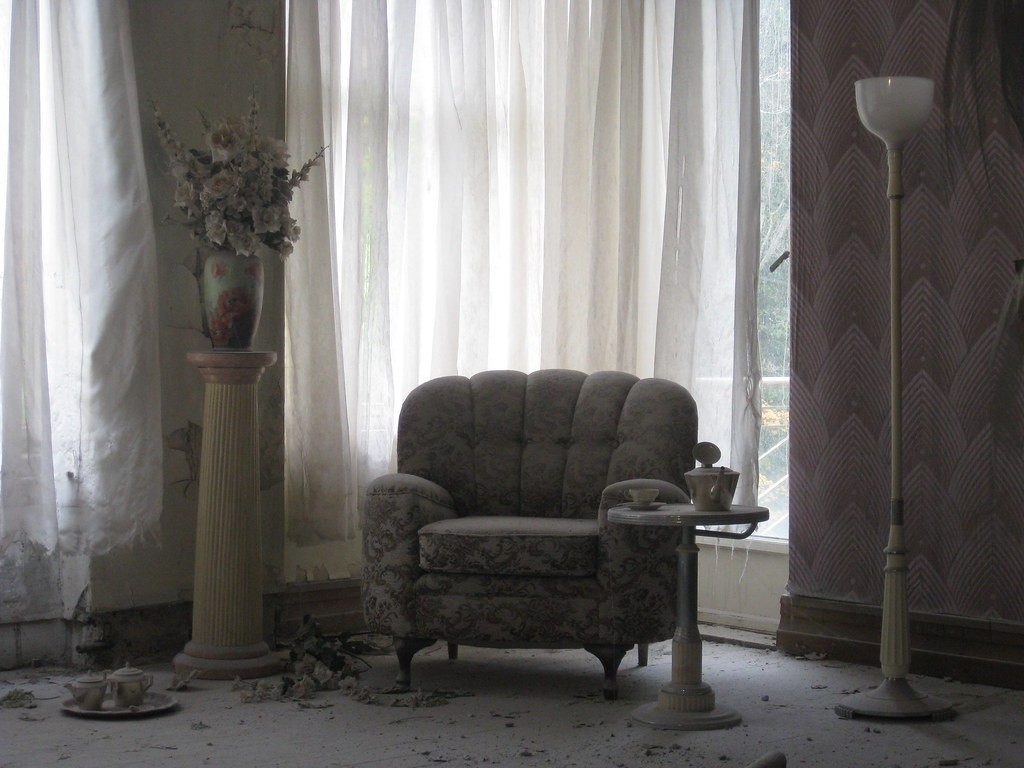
[{"x1": 623, "y1": 488, "x2": 659, "y2": 504}]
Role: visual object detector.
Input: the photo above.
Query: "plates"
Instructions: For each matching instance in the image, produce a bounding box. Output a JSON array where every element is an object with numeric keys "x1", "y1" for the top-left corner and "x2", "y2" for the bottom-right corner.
[
  {"x1": 61, "y1": 692, "x2": 178, "y2": 718},
  {"x1": 620, "y1": 502, "x2": 666, "y2": 510}
]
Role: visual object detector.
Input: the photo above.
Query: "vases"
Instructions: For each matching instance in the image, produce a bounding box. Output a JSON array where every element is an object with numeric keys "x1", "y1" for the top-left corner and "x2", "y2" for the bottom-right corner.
[{"x1": 201, "y1": 235, "x2": 266, "y2": 351}]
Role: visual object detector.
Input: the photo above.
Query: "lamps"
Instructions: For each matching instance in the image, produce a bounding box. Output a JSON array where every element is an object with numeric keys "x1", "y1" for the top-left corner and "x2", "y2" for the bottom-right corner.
[{"x1": 834, "y1": 75, "x2": 960, "y2": 723}]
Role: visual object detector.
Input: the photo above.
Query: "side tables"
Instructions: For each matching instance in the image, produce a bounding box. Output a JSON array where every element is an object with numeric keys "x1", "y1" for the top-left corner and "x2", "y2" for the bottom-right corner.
[{"x1": 605, "y1": 501, "x2": 771, "y2": 735}]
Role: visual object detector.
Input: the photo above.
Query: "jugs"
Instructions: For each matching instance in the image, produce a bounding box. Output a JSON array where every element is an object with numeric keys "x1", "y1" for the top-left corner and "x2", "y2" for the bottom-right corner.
[
  {"x1": 684, "y1": 442, "x2": 740, "y2": 511},
  {"x1": 105, "y1": 661, "x2": 154, "y2": 708},
  {"x1": 64, "y1": 668, "x2": 110, "y2": 711}
]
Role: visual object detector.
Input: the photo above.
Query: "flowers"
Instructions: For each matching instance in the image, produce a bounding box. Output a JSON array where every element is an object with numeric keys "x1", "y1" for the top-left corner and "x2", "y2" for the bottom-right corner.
[{"x1": 149, "y1": 95, "x2": 333, "y2": 263}]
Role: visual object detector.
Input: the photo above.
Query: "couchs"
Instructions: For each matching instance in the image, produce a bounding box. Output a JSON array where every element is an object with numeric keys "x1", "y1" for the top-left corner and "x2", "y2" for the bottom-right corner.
[{"x1": 361, "y1": 366, "x2": 701, "y2": 697}]
